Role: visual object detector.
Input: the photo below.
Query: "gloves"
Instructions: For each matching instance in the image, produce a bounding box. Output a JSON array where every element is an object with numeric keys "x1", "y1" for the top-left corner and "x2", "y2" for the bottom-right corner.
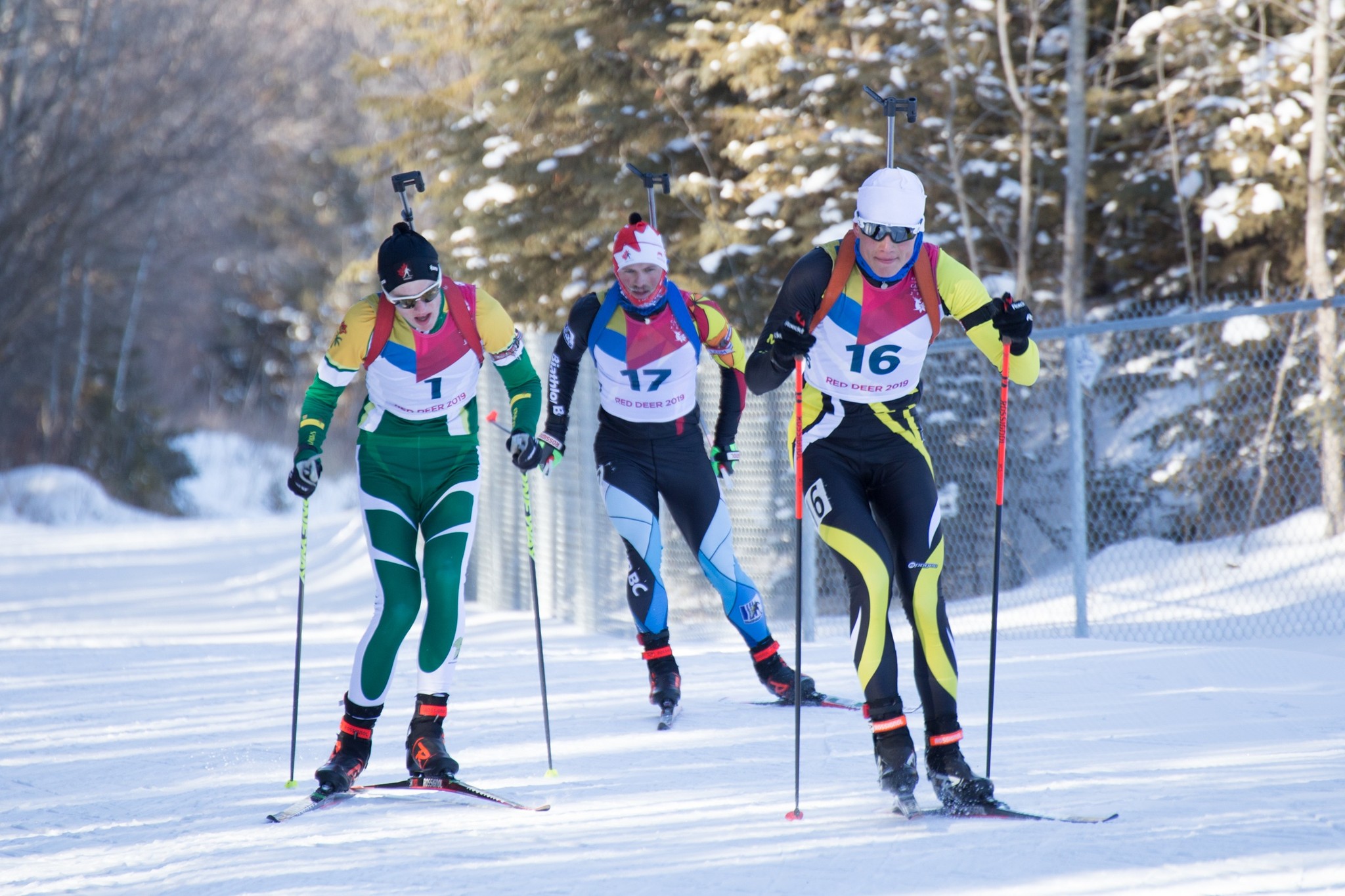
[
  {"x1": 992, "y1": 292, "x2": 1033, "y2": 356},
  {"x1": 506, "y1": 429, "x2": 542, "y2": 468},
  {"x1": 772, "y1": 316, "x2": 817, "y2": 367},
  {"x1": 288, "y1": 457, "x2": 324, "y2": 499},
  {"x1": 536, "y1": 430, "x2": 568, "y2": 476},
  {"x1": 710, "y1": 441, "x2": 737, "y2": 479}
]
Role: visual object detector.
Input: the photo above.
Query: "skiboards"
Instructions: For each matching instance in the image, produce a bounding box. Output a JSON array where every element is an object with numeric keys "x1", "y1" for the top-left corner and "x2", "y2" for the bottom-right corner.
[
  {"x1": 266, "y1": 774, "x2": 552, "y2": 823},
  {"x1": 654, "y1": 694, "x2": 928, "y2": 731},
  {"x1": 887, "y1": 802, "x2": 1120, "y2": 824}
]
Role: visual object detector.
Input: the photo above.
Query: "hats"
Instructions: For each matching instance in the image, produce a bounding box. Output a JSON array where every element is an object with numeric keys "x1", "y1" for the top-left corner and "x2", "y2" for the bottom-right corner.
[
  {"x1": 852, "y1": 169, "x2": 926, "y2": 234},
  {"x1": 611, "y1": 211, "x2": 669, "y2": 308},
  {"x1": 377, "y1": 222, "x2": 440, "y2": 294}
]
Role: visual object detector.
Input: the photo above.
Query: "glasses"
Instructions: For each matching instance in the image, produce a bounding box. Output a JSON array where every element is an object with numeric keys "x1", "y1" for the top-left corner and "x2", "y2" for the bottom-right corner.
[
  {"x1": 857, "y1": 217, "x2": 917, "y2": 243},
  {"x1": 381, "y1": 266, "x2": 443, "y2": 309}
]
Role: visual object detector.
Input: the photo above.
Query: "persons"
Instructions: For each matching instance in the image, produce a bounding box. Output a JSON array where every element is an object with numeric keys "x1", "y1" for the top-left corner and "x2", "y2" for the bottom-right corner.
[
  {"x1": 529, "y1": 209, "x2": 816, "y2": 702},
  {"x1": 287, "y1": 221, "x2": 542, "y2": 794},
  {"x1": 744, "y1": 167, "x2": 1040, "y2": 808}
]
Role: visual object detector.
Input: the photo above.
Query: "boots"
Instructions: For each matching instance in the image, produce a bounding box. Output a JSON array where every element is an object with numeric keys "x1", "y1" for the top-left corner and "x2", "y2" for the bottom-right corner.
[
  {"x1": 637, "y1": 629, "x2": 682, "y2": 705},
  {"x1": 405, "y1": 693, "x2": 459, "y2": 777},
  {"x1": 317, "y1": 689, "x2": 386, "y2": 791},
  {"x1": 748, "y1": 638, "x2": 813, "y2": 701},
  {"x1": 924, "y1": 727, "x2": 992, "y2": 808},
  {"x1": 861, "y1": 697, "x2": 917, "y2": 795}
]
